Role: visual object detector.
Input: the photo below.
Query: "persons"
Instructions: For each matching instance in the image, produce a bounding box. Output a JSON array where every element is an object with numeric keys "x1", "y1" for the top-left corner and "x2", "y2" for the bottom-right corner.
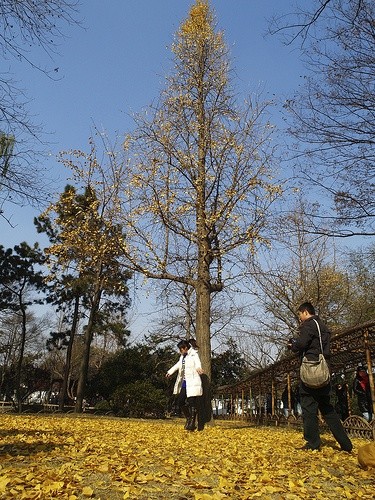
[
  {"x1": 290, "y1": 302, "x2": 354, "y2": 451},
  {"x1": 353, "y1": 366, "x2": 373, "y2": 422},
  {"x1": 266, "y1": 391, "x2": 277, "y2": 416},
  {"x1": 165, "y1": 338, "x2": 204, "y2": 430},
  {"x1": 254, "y1": 392, "x2": 265, "y2": 415},
  {"x1": 227, "y1": 400, "x2": 235, "y2": 414},
  {"x1": 283, "y1": 385, "x2": 296, "y2": 417},
  {"x1": 336, "y1": 383, "x2": 349, "y2": 422}
]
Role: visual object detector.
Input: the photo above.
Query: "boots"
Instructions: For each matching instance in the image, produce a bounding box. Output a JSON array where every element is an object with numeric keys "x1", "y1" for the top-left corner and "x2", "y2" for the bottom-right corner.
[
  {"x1": 186, "y1": 405, "x2": 197, "y2": 431},
  {"x1": 181, "y1": 406, "x2": 191, "y2": 430}
]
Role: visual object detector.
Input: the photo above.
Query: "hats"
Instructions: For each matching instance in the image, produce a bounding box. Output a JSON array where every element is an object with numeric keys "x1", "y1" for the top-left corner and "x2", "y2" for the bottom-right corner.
[{"x1": 357, "y1": 366, "x2": 367, "y2": 373}]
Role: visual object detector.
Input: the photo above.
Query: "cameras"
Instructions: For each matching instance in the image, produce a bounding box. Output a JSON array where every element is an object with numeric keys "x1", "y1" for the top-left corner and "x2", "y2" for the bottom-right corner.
[{"x1": 286, "y1": 337, "x2": 297, "y2": 350}]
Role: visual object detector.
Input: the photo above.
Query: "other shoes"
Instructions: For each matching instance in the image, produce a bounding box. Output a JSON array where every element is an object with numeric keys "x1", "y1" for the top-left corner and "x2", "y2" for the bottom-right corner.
[{"x1": 295, "y1": 443, "x2": 321, "y2": 453}]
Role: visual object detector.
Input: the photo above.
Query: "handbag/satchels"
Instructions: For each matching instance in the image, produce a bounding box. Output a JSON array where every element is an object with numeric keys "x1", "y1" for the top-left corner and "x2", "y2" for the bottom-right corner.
[{"x1": 299, "y1": 355, "x2": 331, "y2": 389}]
